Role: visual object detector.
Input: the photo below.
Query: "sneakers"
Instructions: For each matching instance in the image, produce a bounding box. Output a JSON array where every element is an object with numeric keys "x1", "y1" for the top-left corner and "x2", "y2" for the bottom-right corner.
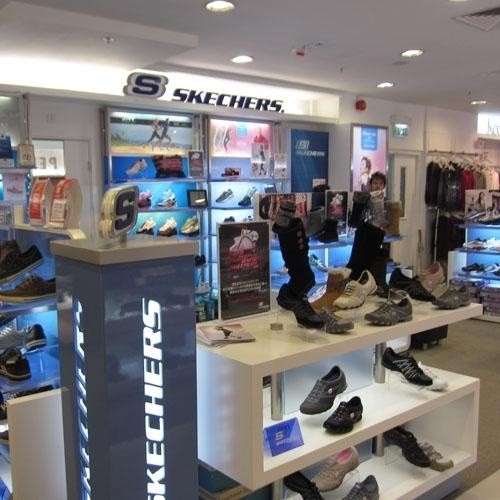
[
  {"x1": 462, "y1": 260, "x2": 500, "y2": 278},
  {"x1": 461, "y1": 209, "x2": 500, "y2": 225},
  {"x1": 1, "y1": 276, "x2": 56, "y2": 303},
  {"x1": 195, "y1": 281, "x2": 211, "y2": 294},
  {"x1": 0, "y1": 324, "x2": 48, "y2": 382},
  {"x1": 124, "y1": 158, "x2": 147, "y2": 176},
  {"x1": 0, "y1": 239, "x2": 44, "y2": 284},
  {"x1": 299, "y1": 366, "x2": 347, "y2": 414},
  {"x1": 324, "y1": 396, "x2": 363, "y2": 432},
  {"x1": 216, "y1": 190, "x2": 234, "y2": 203},
  {"x1": 462, "y1": 238, "x2": 500, "y2": 252},
  {"x1": 383, "y1": 427, "x2": 453, "y2": 471},
  {"x1": 381, "y1": 347, "x2": 448, "y2": 392},
  {"x1": 238, "y1": 186, "x2": 257, "y2": 205},
  {"x1": 284, "y1": 447, "x2": 379, "y2": 500},
  {"x1": 195, "y1": 255, "x2": 206, "y2": 265},
  {"x1": 135, "y1": 188, "x2": 199, "y2": 237},
  {"x1": 365, "y1": 261, "x2": 470, "y2": 326}
]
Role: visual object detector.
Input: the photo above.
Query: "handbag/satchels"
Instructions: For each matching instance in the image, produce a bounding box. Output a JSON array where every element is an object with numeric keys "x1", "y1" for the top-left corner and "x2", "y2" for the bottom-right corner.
[{"x1": 152, "y1": 154, "x2": 187, "y2": 178}]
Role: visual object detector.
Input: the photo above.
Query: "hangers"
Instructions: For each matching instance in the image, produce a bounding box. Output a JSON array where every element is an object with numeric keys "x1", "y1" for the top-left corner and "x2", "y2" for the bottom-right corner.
[{"x1": 427, "y1": 150, "x2": 494, "y2": 169}]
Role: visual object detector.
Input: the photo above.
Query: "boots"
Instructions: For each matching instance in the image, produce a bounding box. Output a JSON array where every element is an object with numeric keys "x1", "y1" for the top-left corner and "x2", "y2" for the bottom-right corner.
[{"x1": 272, "y1": 189, "x2": 400, "y2": 333}]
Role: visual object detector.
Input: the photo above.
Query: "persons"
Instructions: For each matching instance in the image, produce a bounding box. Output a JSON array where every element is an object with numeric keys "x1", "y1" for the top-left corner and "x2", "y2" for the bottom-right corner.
[
  {"x1": 147, "y1": 119, "x2": 161, "y2": 144},
  {"x1": 259, "y1": 145, "x2": 268, "y2": 176},
  {"x1": 358, "y1": 157, "x2": 371, "y2": 192},
  {"x1": 157, "y1": 118, "x2": 172, "y2": 144},
  {"x1": 475, "y1": 192, "x2": 486, "y2": 209},
  {"x1": 369, "y1": 172, "x2": 389, "y2": 193}
]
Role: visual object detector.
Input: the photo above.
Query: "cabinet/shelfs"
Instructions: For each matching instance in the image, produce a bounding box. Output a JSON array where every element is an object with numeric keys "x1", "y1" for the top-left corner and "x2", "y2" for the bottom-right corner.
[
  {"x1": 197, "y1": 291, "x2": 481, "y2": 500},
  {"x1": 446, "y1": 223, "x2": 500, "y2": 322},
  {"x1": 204, "y1": 115, "x2": 276, "y2": 300},
  {"x1": 0, "y1": 169, "x2": 84, "y2": 500},
  {"x1": 269, "y1": 237, "x2": 406, "y2": 285},
  {"x1": 100, "y1": 106, "x2": 208, "y2": 307}
]
